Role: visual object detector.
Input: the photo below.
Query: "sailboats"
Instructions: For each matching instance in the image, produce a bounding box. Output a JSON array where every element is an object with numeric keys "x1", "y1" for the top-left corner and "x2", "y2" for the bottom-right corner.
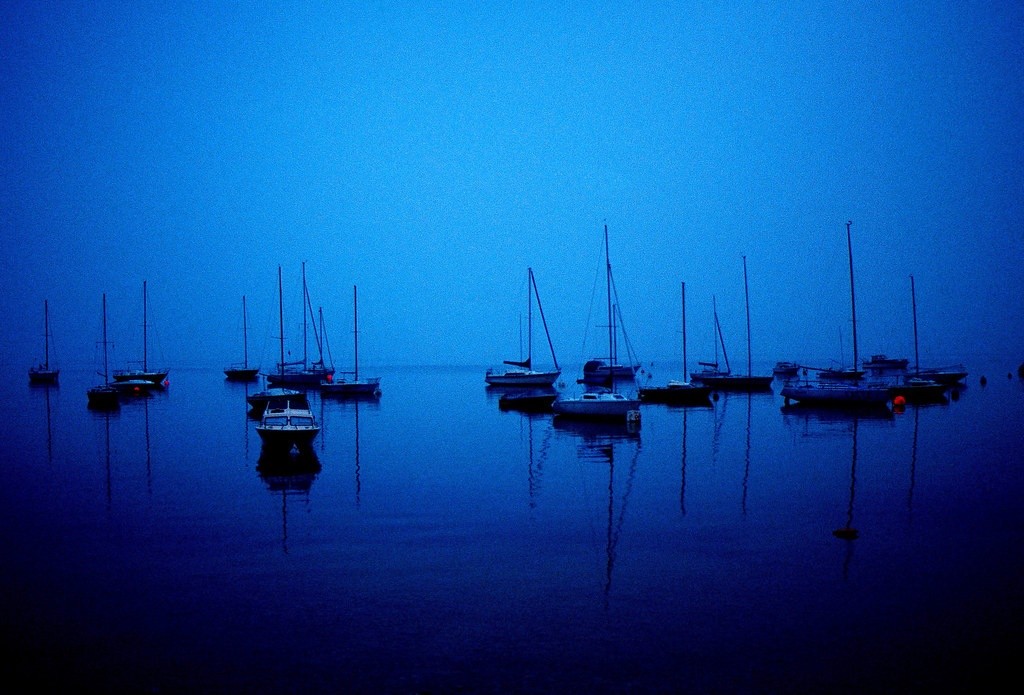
[
  {"x1": 779, "y1": 220, "x2": 970, "y2": 410},
  {"x1": 576, "y1": 302, "x2": 642, "y2": 381},
  {"x1": 692, "y1": 295, "x2": 731, "y2": 380},
  {"x1": 691, "y1": 256, "x2": 779, "y2": 387},
  {"x1": 27, "y1": 298, "x2": 62, "y2": 382},
  {"x1": 221, "y1": 295, "x2": 261, "y2": 379},
  {"x1": 85, "y1": 277, "x2": 173, "y2": 406},
  {"x1": 636, "y1": 281, "x2": 713, "y2": 399},
  {"x1": 267, "y1": 260, "x2": 383, "y2": 395},
  {"x1": 547, "y1": 220, "x2": 644, "y2": 415},
  {"x1": 483, "y1": 266, "x2": 564, "y2": 385}
]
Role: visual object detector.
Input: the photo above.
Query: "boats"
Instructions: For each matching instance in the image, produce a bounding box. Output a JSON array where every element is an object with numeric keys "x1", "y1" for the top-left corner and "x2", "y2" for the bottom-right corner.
[
  {"x1": 769, "y1": 359, "x2": 802, "y2": 376},
  {"x1": 245, "y1": 389, "x2": 307, "y2": 408},
  {"x1": 254, "y1": 397, "x2": 322, "y2": 441}
]
